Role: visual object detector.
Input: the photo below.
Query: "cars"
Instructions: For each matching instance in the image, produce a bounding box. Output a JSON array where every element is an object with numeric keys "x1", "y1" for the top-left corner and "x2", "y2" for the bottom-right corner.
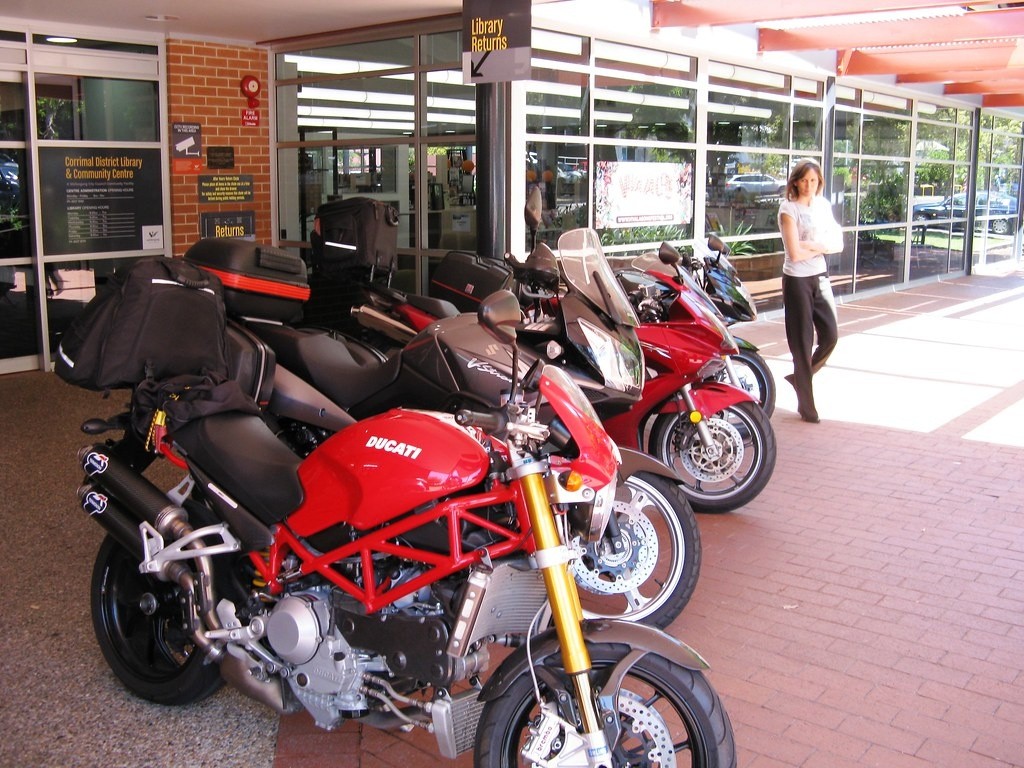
[
  {"x1": 912, "y1": 191, "x2": 1017, "y2": 235},
  {"x1": 525, "y1": 152, "x2": 587, "y2": 184}
]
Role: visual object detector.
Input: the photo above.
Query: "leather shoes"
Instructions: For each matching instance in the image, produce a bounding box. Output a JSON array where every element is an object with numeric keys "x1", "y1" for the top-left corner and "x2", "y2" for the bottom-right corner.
[{"x1": 799, "y1": 410, "x2": 820, "y2": 423}]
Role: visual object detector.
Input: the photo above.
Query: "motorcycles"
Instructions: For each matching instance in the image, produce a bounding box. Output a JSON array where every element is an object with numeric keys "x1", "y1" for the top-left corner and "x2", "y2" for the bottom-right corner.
[
  {"x1": 182, "y1": 187, "x2": 702, "y2": 631},
  {"x1": 428, "y1": 235, "x2": 776, "y2": 438},
  {"x1": 309, "y1": 197, "x2": 777, "y2": 515},
  {"x1": 54, "y1": 256, "x2": 735, "y2": 768}
]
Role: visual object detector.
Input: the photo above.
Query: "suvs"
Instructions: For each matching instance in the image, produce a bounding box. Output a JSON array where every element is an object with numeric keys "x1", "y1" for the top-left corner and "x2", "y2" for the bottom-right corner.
[{"x1": 724, "y1": 174, "x2": 787, "y2": 195}]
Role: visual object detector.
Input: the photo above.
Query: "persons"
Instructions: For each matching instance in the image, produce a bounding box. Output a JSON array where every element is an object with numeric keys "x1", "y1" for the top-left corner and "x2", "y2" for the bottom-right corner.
[{"x1": 776, "y1": 159, "x2": 844, "y2": 424}]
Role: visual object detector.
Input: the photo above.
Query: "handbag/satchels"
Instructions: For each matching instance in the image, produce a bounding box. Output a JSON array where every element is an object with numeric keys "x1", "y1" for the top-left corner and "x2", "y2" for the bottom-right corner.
[{"x1": 53, "y1": 257, "x2": 228, "y2": 400}]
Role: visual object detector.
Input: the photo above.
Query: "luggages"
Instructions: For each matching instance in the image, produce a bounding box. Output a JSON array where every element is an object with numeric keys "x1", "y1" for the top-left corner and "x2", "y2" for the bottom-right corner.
[{"x1": 311, "y1": 196, "x2": 399, "y2": 280}]
